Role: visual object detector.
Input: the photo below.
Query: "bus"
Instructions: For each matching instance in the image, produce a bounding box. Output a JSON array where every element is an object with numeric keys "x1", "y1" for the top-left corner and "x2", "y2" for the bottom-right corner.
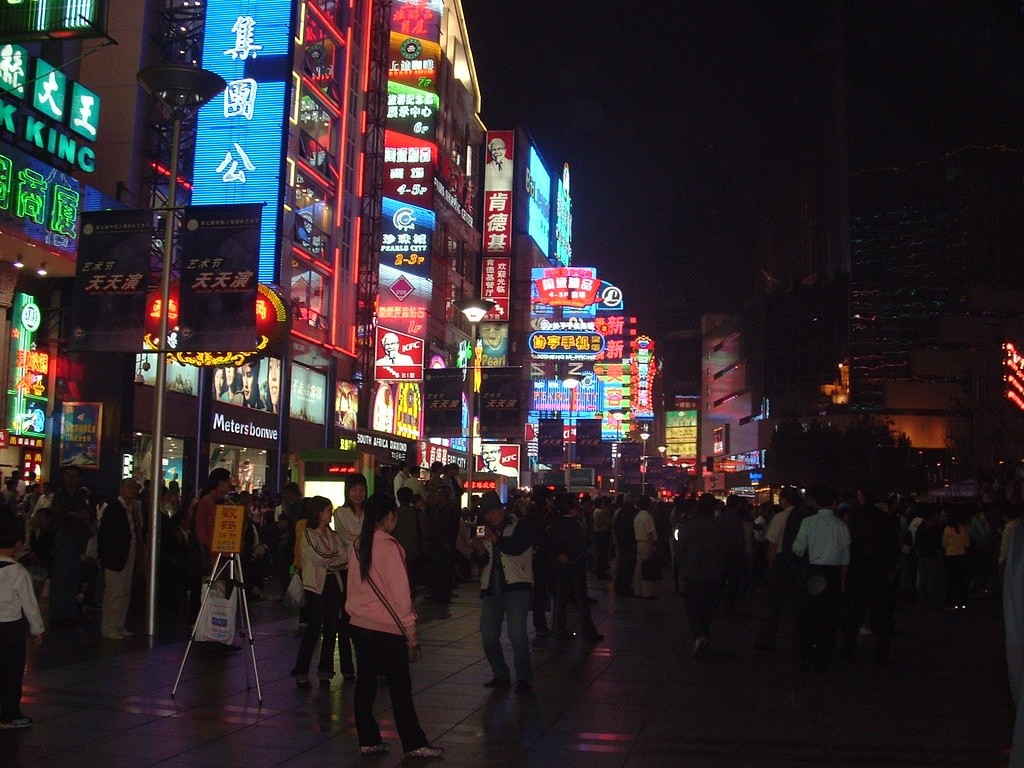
[{"x1": 572, "y1": 469, "x2": 608, "y2": 502}]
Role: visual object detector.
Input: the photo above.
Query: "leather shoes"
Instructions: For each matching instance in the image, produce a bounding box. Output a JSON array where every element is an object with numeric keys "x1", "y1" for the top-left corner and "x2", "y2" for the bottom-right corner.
[
  {"x1": 317, "y1": 675, "x2": 331, "y2": 688},
  {"x1": 293, "y1": 675, "x2": 313, "y2": 691}
]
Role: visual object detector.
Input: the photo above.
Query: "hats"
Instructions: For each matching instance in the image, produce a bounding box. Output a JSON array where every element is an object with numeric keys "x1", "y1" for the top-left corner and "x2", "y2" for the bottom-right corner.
[{"x1": 477, "y1": 490, "x2": 508, "y2": 514}]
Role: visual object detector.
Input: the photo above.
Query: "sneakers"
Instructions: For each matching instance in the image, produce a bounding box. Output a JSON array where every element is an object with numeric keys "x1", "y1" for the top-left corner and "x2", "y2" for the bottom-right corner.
[
  {"x1": 691, "y1": 636, "x2": 711, "y2": 658},
  {"x1": 0, "y1": 714, "x2": 34, "y2": 729},
  {"x1": 360, "y1": 741, "x2": 391, "y2": 754},
  {"x1": 404, "y1": 744, "x2": 445, "y2": 758}
]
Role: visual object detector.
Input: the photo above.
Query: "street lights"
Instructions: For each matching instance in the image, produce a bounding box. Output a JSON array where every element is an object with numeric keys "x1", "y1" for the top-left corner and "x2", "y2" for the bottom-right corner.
[
  {"x1": 670, "y1": 455, "x2": 681, "y2": 499},
  {"x1": 657, "y1": 445, "x2": 668, "y2": 489},
  {"x1": 681, "y1": 462, "x2": 689, "y2": 489},
  {"x1": 608, "y1": 409, "x2": 629, "y2": 502},
  {"x1": 638, "y1": 430, "x2": 652, "y2": 495},
  {"x1": 557, "y1": 374, "x2": 586, "y2": 493},
  {"x1": 454, "y1": 298, "x2": 496, "y2": 543},
  {"x1": 136, "y1": 63, "x2": 228, "y2": 651}
]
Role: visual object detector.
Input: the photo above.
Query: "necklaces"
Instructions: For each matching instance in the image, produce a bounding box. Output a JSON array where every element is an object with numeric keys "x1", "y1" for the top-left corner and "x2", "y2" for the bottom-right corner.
[{"x1": 485, "y1": 338, "x2": 504, "y2": 350}]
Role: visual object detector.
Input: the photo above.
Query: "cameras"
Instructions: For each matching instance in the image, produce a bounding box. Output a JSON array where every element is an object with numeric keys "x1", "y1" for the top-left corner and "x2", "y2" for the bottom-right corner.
[{"x1": 476, "y1": 526, "x2": 485, "y2": 536}]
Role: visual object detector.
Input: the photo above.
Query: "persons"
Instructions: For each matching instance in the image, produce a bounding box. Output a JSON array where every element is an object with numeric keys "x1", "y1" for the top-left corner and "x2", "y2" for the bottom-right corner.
[
  {"x1": 213, "y1": 357, "x2": 281, "y2": 414},
  {"x1": 477, "y1": 320, "x2": 510, "y2": 361},
  {"x1": 338, "y1": 393, "x2": 358, "y2": 430},
  {"x1": 0, "y1": 464, "x2": 1024, "y2": 759}
]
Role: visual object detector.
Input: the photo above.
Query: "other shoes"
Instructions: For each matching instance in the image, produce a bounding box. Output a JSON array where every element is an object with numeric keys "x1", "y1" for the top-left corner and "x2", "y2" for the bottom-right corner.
[
  {"x1": 118, "y1": 630, "x2": 134, "y2": 637},
  {"x1": 101, "y1": 631, "x2": 124, "y2": 640},
  {"x1": 484, "y1": 676, "x2": 511, "y2": 688},
  {"x1": 514, "y1": 683, "x2": 531, "y2": 694}
]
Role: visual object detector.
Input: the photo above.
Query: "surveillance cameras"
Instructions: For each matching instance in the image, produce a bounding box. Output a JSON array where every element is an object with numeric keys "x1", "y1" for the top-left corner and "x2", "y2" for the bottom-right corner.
[
  {"x1": 134, "y1": 374, "x2": 145, "y2": 386},
  {"x1": 142, "y1": 363, "x2": 150, "y2": 371}
]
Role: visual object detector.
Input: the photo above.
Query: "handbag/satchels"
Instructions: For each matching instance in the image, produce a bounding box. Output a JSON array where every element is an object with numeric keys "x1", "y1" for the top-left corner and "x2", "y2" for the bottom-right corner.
[
  {"x1": 281, "y1": 573, "x2": 307, "y2": 609},
  {"x1": 407, "y1": 642, "x2": 421, "y2": 664},
  {"x1": 641, "y1": 551, "x2": 664, "y2": 581},
  {"x1": 195, "y1": 583, "x2": 238, "y2": 645},
  {"x1": 428, "y1": 551, "x2": 467, "y2": 591}
]
